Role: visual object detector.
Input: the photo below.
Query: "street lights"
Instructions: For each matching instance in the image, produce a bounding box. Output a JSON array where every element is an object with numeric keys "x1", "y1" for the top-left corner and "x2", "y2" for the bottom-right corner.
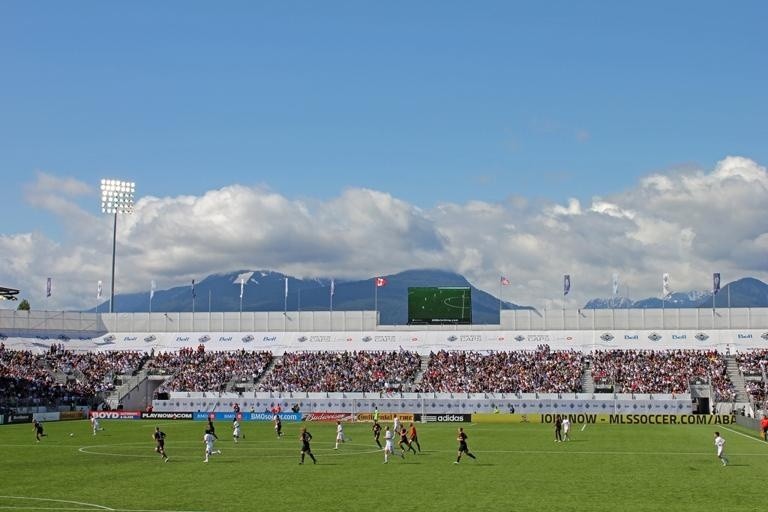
[{"x1": 101, "y1": 179, "x2": 135, "y2": 311}]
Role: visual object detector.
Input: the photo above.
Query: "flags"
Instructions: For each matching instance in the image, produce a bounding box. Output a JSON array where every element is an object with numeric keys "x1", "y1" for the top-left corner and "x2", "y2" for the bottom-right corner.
[
  {"x1": 376, "y1": 276, "x2": 387, "y2": 287},
  {"x1": 501, "y1": 276, "x2": 511, "y2": 286}
]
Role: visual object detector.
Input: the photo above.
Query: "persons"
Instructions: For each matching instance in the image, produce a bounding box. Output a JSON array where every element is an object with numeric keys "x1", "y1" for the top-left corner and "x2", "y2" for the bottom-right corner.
[
  {"x1": 333, "y1": 421, "x2": 352, "y2": 450},
  {"x1": 454, "y1": 428, "x2": 477, "y2": 464},
  {"x1": 1, "y1": 344, "x2": 768, "y2": 418},
  {"x1": 554, "y1": 416, "x2": 570, "y2": 442},
  {"x1": 275, "y1": 416, "x2": 284, "y2": 437},
  {"x1": 299, "y1": 428, "x2": 317, "y2": 464},
  {"x1": 233, "y1": 404, "x2": 245, "y2": 443},
  {"x1": 203, "y1": 417, "x2": 221, "y2": 463},
  {"x1": 714, "y1": 432, "x2": 728, "y2": 464},
  {"x1": 371, "y1": 407, "x2": 421, "y2": 464},
  {"x1": 152, "y1": 427, "x2": 169, "y2": 463},
  {"x1": 33, "y1": 419, "x2": 48, "y2": 442},
  {"x1": 760, "y1": 415, "x2": 768, "y2": 440},
  {"x1": 90, "y1": 416, "x2": 104, "y2": 435}
]
